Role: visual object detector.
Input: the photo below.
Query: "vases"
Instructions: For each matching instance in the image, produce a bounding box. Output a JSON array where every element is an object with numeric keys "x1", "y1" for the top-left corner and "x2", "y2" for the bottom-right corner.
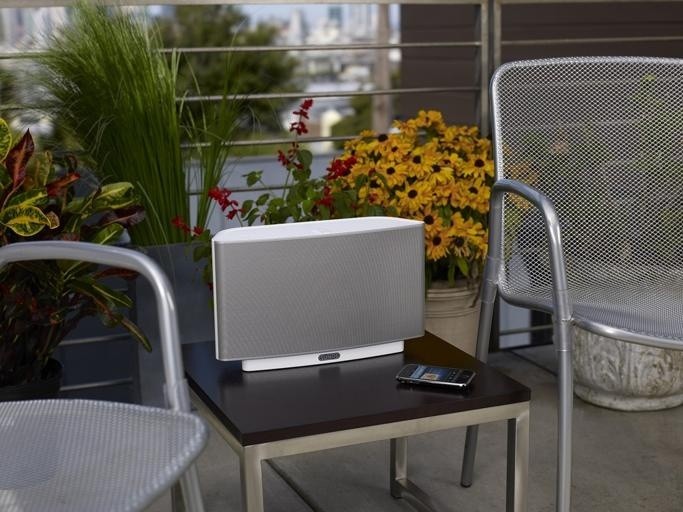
[{"x1": 421, "y1": 277, "x2": 482, "y2": 358}]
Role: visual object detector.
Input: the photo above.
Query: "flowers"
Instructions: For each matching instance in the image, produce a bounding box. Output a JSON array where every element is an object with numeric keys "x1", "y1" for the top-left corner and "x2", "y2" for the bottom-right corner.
[
  {"x1": 322, "y1": 112, "x2": 541, "y2": 306},
  {"x1": 173, "y1": 97, "x2": 393, "y2": 259}
]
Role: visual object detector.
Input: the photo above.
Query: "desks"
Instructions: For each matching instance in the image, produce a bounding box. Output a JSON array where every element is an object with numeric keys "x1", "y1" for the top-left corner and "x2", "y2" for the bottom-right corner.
[{"x1": 171, "y1": 324, "x2": 531, "y2": 512}]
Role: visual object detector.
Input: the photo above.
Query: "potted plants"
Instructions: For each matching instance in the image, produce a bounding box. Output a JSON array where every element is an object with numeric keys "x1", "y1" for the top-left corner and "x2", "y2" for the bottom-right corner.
[
  {"x1": 554, "y1": 69, "x2": 683, "y2": 412},
  {"x1": 0, "y1": 113, "x2": 152, "y2": 402}
]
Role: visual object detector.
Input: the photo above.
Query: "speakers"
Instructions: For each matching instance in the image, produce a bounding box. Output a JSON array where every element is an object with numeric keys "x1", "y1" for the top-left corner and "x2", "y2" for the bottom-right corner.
[{"x1": 211, "y1": 215, "x2": 425, "y2": 372}]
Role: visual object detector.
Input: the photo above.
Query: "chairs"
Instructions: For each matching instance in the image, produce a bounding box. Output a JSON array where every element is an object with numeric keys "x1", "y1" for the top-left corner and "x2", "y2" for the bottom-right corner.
[
  {"x1": 0, "y1": 240, "x2": 207, "y2": 512},
  {"x1": 457, "y1": 55, "x2": 683, "y2": 512}
]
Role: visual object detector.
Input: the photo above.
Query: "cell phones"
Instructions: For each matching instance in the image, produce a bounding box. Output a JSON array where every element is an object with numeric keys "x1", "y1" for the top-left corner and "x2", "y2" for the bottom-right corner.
[{"x1": 395, "y1": 363, "x2": 476, "y2": 389}]
